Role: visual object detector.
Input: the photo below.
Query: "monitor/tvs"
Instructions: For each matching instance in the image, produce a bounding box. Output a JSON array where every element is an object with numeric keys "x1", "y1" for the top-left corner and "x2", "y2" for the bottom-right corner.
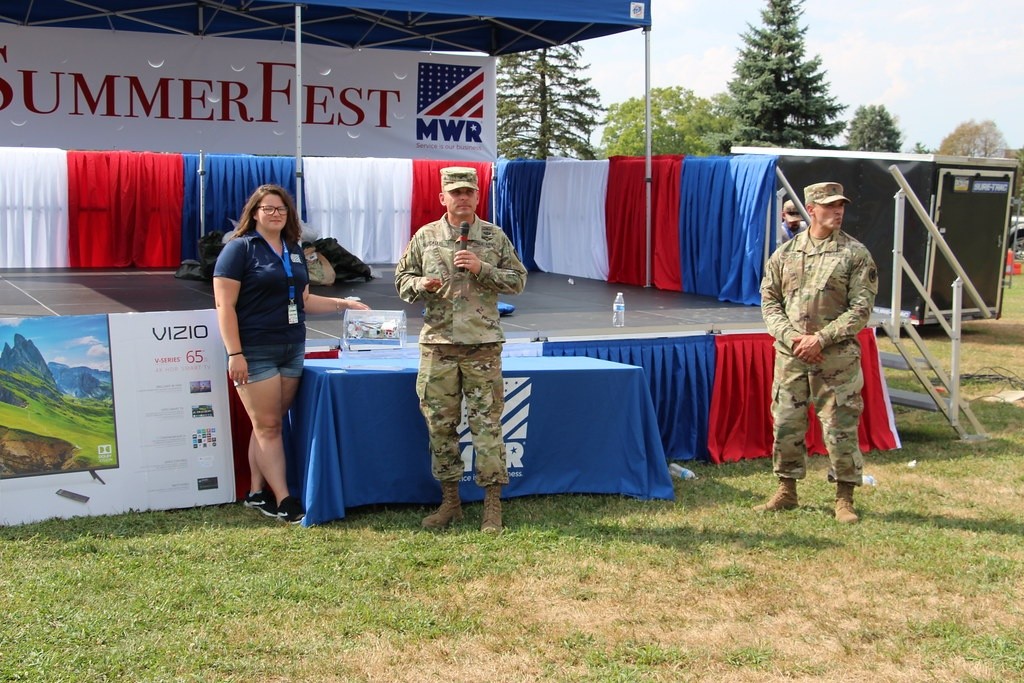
[{"x1": 0, "y1": 314, "x2": 119, "y2": 486}]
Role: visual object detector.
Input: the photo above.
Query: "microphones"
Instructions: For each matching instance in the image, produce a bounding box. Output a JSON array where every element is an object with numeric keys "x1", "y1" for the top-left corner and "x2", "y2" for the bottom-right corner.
[{"x1": 457, "y1": 221, "x2": 470, "y2": 272}]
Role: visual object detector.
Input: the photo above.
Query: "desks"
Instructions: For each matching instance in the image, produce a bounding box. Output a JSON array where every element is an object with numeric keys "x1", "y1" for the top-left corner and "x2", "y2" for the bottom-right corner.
[{"x1": 281, "y1": 356, "x2": 676, "y2": 529}]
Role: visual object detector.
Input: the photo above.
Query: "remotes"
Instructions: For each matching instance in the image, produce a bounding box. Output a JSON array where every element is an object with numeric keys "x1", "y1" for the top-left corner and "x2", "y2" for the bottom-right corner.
[{"x1": 56, "y1": 488, "x2": 90, "y2": 502}]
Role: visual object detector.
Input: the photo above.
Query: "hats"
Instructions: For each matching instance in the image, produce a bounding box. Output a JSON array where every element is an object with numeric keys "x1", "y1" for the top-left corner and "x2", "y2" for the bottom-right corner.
[
  {"x1": 783, "y1": 200, "x2": 805, "y2": 223},
  {"x1": 440, "y1": 167, "x2": 480, "y2": 192},
  {"x1": 805, "y1": 181, "x2": 851, "y2": 205}
]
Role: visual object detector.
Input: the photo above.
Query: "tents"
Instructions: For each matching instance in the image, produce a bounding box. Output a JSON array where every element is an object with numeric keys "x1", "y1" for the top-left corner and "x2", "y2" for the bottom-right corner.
[{"x1": 0, "y1": 0, "x2": 653, "y2": 290}]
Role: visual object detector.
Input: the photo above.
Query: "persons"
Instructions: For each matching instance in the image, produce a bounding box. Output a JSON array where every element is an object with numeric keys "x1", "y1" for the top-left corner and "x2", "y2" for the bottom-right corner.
[
  {"x1": 782, "y1": 199, "x2": 808, "y2": 244},
  {"x1": 213, "y1": 185, "x2": 373, "y2": 525},
  {"x1": 752, "y1": 182, "x2": 881, "y2": 525},
  {"x1": 394, "y1": 166, "x2": 528, "y2": 535}
]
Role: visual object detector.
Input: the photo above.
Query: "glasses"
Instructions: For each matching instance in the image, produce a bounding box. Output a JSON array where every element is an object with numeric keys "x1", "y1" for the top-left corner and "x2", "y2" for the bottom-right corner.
[{"x1": 258, "y1": 205, "x2": 289, "y2": 216}]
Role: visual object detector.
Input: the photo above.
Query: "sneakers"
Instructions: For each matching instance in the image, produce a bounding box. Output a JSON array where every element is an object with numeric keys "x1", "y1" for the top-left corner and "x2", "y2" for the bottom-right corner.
[
  {"x1": 277, "y1": 495, "x2": 306, "y2": 525},
  {"x1": 244, "y1": 491, "x2": 279, "y2": 517}
]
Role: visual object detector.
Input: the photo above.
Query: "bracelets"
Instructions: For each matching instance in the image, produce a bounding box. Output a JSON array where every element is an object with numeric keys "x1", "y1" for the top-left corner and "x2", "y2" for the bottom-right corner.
[
  {"x1": 337, "y1": 298, "x2": 340, "y2": 311},
  {"x1": 229, "y1": 352, "x2": 244, "y2": 356}
]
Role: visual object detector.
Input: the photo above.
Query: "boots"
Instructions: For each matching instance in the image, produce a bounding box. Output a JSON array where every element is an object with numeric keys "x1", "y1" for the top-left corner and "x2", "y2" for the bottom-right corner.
[
  {"x1": 751, "y1": 479, "x2": 799, "y2": 514},
  {"x1": 481, "y1": 484, "x2": 502, "y2": 535},
  {"x1": 834, "y1": 481, "x2": 858, "y2": 524},
  {"x1": 422, "y1": 484, "x2": 464, "y2": 528}
]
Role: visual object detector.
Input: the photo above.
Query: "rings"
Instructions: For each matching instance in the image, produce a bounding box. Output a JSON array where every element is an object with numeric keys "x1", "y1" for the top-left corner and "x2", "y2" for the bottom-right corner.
[
  {"x1": 433, "y1": 287, "x2": 437, "y2": 291},
  {"x1": 467, "y1": 260, "x2": 468, "y2": 263},
  {"x1": 803, "y1": 357, "x2": 806, "y2": 361}
]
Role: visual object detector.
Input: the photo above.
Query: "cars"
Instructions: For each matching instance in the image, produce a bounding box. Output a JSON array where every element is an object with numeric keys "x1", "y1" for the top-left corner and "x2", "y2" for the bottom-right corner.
[{"x1": 1008, "y1": 223, "x2": 1024, "y2": 260}]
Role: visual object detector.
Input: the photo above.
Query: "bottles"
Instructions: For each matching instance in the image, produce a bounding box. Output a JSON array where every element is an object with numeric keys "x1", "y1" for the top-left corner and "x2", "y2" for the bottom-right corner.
[
  {"x1": 612, "y1": 291, "x2": 625, "y2": 328},
  {"x1": 668, "y1": 462, "x2": 700, "y2": 480},
  {"x1": 860, "y1": 473, "x2": 877, "y2": 485}
]
